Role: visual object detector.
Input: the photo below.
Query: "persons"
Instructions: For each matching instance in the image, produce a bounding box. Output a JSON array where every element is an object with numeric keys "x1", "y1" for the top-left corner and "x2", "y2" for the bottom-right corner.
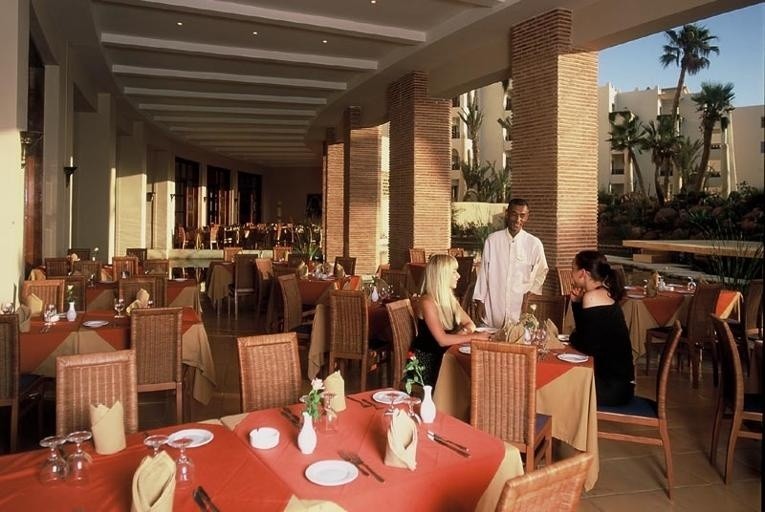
[
  {"x1": 569, "y1": 249, "x2": 635, "y2": 408},
  {"x1": 408, "y1": 253, "x2": 491, "y2": 385},
  {"x1": 472, "y1": 199, "x2": 550, "y2": 329}
]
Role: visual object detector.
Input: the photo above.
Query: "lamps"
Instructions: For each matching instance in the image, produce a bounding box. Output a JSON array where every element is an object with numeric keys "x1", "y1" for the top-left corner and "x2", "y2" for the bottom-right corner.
[
  {"x1": 170, "y1": 194, "x2": 176, "y2": 202},
  {"x1": 204, "y1": 197, "x2": 209, "y2": 201},
  {"x1": 146, "y1": 192, "x2": 156, "y2": 202},
  {"x1": 20, "y1": 131, "x2": 44, "y2": 168},
  {"x1": 64, "y1": 166, "x2": 79, "y2": 188}
]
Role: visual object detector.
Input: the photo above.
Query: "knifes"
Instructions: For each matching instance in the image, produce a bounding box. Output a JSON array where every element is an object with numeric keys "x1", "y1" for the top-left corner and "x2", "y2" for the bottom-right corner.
[
  {"x1": 283, "y1": 405, "x2": 304, "y2": 425},
  {"x1": 429, "y1": 430, "x2": 470, "y2": 452},
  {"x1": 427, "y1": 434, "x2": 471, "y2": 458},
  {"x1": 198, "y1": 485, "x2": 220, "y2": 512},
  {"x1": 193, "y1": 489, "x2": 210, "y2": 512},
  {"x1": 281, "y1": 411, "x2": 301, "y2": 428}
]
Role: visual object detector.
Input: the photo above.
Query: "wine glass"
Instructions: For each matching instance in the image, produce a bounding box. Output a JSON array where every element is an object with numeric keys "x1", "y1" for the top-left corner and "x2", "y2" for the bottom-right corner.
[
  {"x1": 65, "y1": 430, "x2": 93, "y2": 488},
  {"x1": 404, "y1": 397, "x2": 422, "y2": 432},
  {"x1": 113, "y1": 297, "x2": 125, "y2": 318},
  {"x1": 44, "y1": 303, "x2": 57, "y2": 326},
  {"x1": 39, "y1": 435, "x2": 69, "y2": 487},
  {"x1": 143, "y1": 435, "x2": 167, "y2": 455},
  {"x1": 686, "y1": 275, "x2": 694, "y2": 289},
  {"x1": 317, "y1": 392, "x2": 338, "y2": 432},
  {"x1": 172, "y1": 437, "x2": 197, "y2": 488},
  {"x1": 643, "y1": 278, "x2": 649, "y2": 293},
  {"x1": 1, "y1": 303, "x2": 12, "y2": 314},
  {"x1": 382, "y1": 394, "x2": 400, "y2": 439},
  {"x1": 379, "y1": 287, "x2": 389, "y2": 299}
]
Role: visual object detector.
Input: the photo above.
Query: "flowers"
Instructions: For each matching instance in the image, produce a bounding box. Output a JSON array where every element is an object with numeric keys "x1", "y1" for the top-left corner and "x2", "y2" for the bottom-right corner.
[
  {"x1": 524, "y1": 304, "x2": 538, "y2": 328},
  {"x1": 402, "y1": 351, "x2": 427, "y2": 383},
  {"x1": 370, "y1": 273, "x2": 380, "y2": 286},
  {"x1": 299, "y1": 378, "x2": 323, "y2": 415},
  {"x1": 66, "y1": 284, "x2": 74, "y2": 300}
]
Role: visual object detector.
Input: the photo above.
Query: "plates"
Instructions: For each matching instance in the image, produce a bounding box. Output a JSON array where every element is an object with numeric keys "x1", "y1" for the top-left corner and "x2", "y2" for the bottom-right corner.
[
  {"x1": 458, "y1": 346, "x2": 471, "y2": 354},
  {"x1": 83, "y1": 320, "x2": 110, "y2": 328},
  {"x1": 304, "y1": 459, "x2": 359, "y2": 488},
  {"x1": 476, "y1": 328, "x2": 499, "y2": 334},
  {"x1": 556, "y1": 353, "x2": 590, "y2": 363},
  {"x1": 627, "y1": 295, "x2": 645, "y2": 299},
  {"x1": 668, "y1": 284, "x2": 683, "y2": 287},
  {"x1": 59, "y1": 311, "x2": 67, "y2": 319},
  {"x1": 624, "y1": 286, "x2": 636, "y2": 290},
  {"x1": 557, "y1": 335, "x2": 570, "y2": 341},
  {"x1": 167, "y1": 428, "x2": 214, "y2": 448},
  {"x1": 372, "y1": 390, "x2": 409, "y2": 405},
  {"x1": 677, "y1": 290, "x2": 691, "y2": 294}
]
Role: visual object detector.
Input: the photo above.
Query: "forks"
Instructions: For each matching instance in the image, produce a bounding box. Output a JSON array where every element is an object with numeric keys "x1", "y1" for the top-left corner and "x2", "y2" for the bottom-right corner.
[
  {"x1": 337, "y1": 449, "x2": 369, "y2": 478},
  {"x1": 351, "y1": 452, "x2": 385, "y2": 484},
  {"x1": 361, "y1": 398, "x2": 385, "y2": 410},
  {"x1": 347, "y1": 397, "x2": 373, "y2": 408}
]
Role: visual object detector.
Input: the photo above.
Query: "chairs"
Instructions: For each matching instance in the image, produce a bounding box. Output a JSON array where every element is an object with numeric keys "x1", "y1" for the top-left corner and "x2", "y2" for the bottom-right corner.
[
  {"x1": 557, "y1": 267, "x2": 580, "y2": 295},
  {"x1": 287, "y1": 253, "x2": 312, "y2": 265},
  {"x1": 112, "y1": 257, "x2": 138, "y2": 282},
  {"x1": 333, "y1": 257, "x2": 357, "y2": 277},
  {"x1": 45, "y1": 258, "x2": 70, "y2": 277},
  {"x1": 255, "y1": 257, "x2": 274, "y2": 317},
  {"x1": 386, "y1": 300, "x2": 427, "y2": 395},
  {"x1": 278, "y1": 274, "x2": 325, "y2": 381},
  {"x1": 296, "y1": 227, "x2": 321, "y2": 246},
  {"x1": 178, "y1": 225, "x2": 189, "y2": 249},
  {"x1": 273, "y1": 246, "x2": 292, "y2": 261},
  {"x1": 223, "y1": 247, "x2": 243, "y2": 262},
  {"x1": 55, "y1": 348, "x2": 138, "y2": 437},
  {"x1": 657, "y1": 337, "x2": 716, "y2": 373},
  {"x1": 559, "y1": 321, "x2": 683, "y2": 500},
  {"x1": 46, "y1": 276, "x2": 87, "y2": 312},
  {"x1": 469, "y1": 339, "x2": 553, "y2": 472},
  {"x1": 228, "y1": 254, "x2": 259, "y2": 316},
  {"x1": 708, "y1": 314, "x2": 763, "y2": 485},
  {"x1": 242, "y1": 223, "x2": 295, "y2": 249},
  {"x1": 719, "y1": 279, "x2": 764, "y2": 378},
  {"x1": 380, "y1": 269, "x2": 408, "y2": 294},
  {"x1": 204, "y1": 223, "x2": 220, "y2": 249},
  {"x1": 119, "y1": 276, "x2": 168, "y2": 307},
  {"x1": 376, "y1": 264, "x2": 390, "y2": 272},
  {"x1": 619, "y1": 274, "x2": 657, "y2": 286},
  {"x1": 236, "y1": 332, "x2": 304, "y2": 414},
  {"x1": 410, "y1": 249, "x2": 426, "y2": 262},
  {"x1": 133, "y1": 308, "x2": 183, "y2": 428},
  {"x1": 447, "y1": 248, "x2": 465, "y2": 258},
  {"x1": 520, "y1": 293, "x2": 566, "y2": 323},
  {"x1": 611, "y1": 265, "x2": 629, "y2": 290},
  {"x1": 126, "y1": 248, "x2": 146, "y2": 265},
  {"x1": 143, "y1": 259, "x2": 170, "y2": 279},
  {"x1": 0, "y1": 315, "x2": 19, "y2": 457},
  {"x1": 646, "y1": 282, "x2": 720, "y2": 389},
  {"x1": 328, "y1": 289, "x2": 378, "y2": 391},
  {"x1": 67, "y1": 248, "x2": 90, "y2": 260},
  {"x1": 73, "y1": 261, "x2": 102, "y2": 280},
  {"x1": 22, "y1": 279, "x2": 66, "y2": 318}
]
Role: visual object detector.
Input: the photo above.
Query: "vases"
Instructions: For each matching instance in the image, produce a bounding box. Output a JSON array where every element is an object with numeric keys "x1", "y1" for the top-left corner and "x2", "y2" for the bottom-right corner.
[
  {"x1": 420, "y1": 385, "x2": 438, "y2": 425},
  {"x1": 297, "y1": 412, "x2": 318, "y2": 455},
  {"x1": 371, "y1": 288, "x2": 379, "y2": 302},
  {"x1": 67, "y1": 302, "x2": 77, "y2": 321},
  {"x1": 520, "y1": 329, "x2": 531, "y2": 344}
]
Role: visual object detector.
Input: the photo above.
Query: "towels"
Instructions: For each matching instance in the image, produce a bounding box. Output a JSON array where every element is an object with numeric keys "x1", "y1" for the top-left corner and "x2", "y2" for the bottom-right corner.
[
  {"x1": 88, "y1": 401, "x2": 127, "y2": 456},
  {"x1": 16, "y1": 304, "x2": 32, "y2": 333},
  {"x1": 545, "y1": 319, "x2": 563, "y2": 349},
  {"x1": 336, "y1": 263, "x2": 344, "y2": 277},
  {"x1": 324, "y1": 371, "x2": 347, "y2": 414},
  {"x1": 492, "y1": 325, "x2": 513, "y2": 342},
  {"x1": 508, "y1": 326, "x2": 532, "y2": 346},
  {"x1": 131, "y1": 451, "x2": 177, "y2": 512},
  {"x1": 27, "y1": 294, "x2": 42, "y2": 318},
  {"x1": 383, "y1": 408, "x2": 417, "y2": 472},
  {"x1": 284, "y1": 495, "x2": 344, "y2": 512},
  {"x1": 298, "y1": 260, "x2": 305, "y2": 270},
  {"x1": 127, "y1": 300, "x2": 143, "y2": 317},
  {"x1": 300, "y1": 264, "x2": 309, "y2": 282},
  {"x1": 137, "y1": 289, "x2": 149, "y2": 309}
]
